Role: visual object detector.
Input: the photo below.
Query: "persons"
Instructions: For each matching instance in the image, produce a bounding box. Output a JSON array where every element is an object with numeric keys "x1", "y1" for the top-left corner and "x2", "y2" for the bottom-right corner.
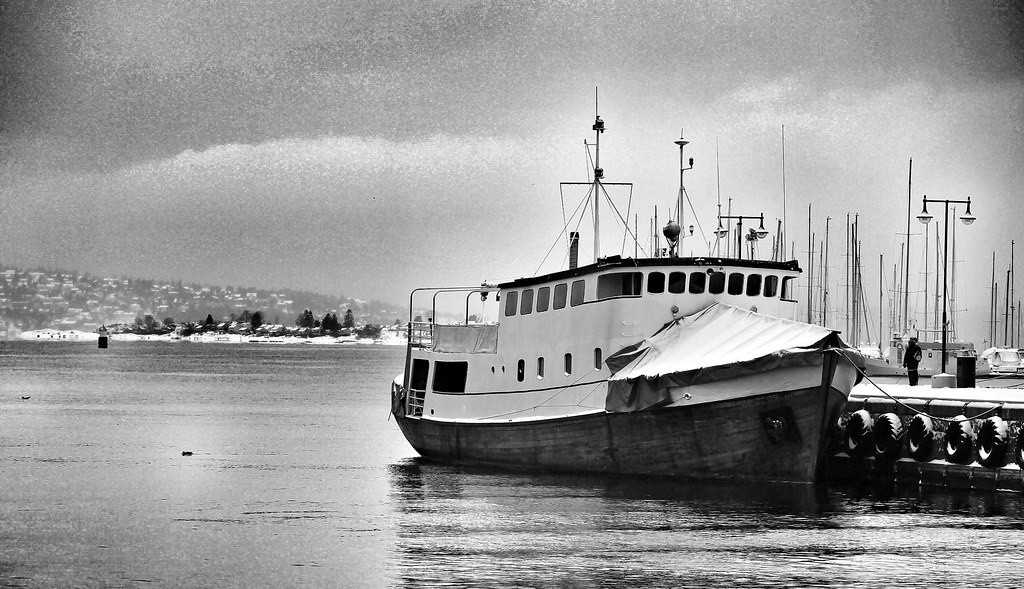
[{"x1": 903, "y1": 338, "x2": 922, "y2": 386}]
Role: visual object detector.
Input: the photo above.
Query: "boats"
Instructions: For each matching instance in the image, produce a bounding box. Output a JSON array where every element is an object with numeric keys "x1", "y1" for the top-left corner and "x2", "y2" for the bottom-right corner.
[{"x1": 387, "y1": 86, "x2": 866, "y2": 487}]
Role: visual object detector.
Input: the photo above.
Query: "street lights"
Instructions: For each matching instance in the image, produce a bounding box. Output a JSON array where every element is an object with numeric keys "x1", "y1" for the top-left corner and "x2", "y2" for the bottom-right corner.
[
  {"x1": 915, "y1": 194, "x2": 977, "y2": 372},
  {"x1": 713, "y1": 212, "x2": 770, "y2": 260}
]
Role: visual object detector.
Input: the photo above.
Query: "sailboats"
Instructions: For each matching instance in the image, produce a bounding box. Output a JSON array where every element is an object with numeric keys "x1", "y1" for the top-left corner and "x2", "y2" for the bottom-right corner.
[{"x1": 632, "y1": 122, "x2": 1024, "y2": 380}]
